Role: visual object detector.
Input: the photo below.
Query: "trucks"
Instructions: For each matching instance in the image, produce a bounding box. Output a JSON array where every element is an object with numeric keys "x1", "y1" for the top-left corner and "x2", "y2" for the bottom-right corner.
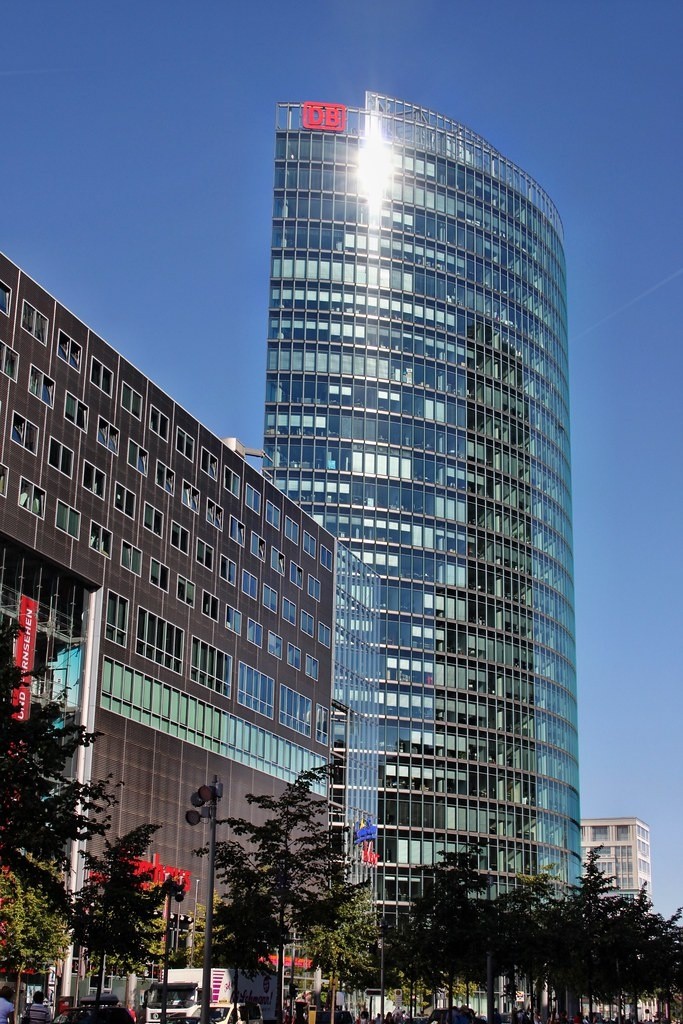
[{"x1": 143, "y1": 965, "x2": 279, "y2": 1024}]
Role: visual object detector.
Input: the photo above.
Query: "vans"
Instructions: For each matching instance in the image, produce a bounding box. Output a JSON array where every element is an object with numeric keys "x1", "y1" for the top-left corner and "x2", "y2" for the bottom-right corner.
[{"x1": 166, "y1": 999, "x2": 264, "y2": 1024}]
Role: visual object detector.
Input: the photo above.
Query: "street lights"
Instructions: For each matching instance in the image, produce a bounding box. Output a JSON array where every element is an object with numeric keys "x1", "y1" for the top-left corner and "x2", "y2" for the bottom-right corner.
[
  {"x1": 377, "y1": 921, "x2": 394, "y2": 1024},
  {"x1": 160, "y1": 877, "x2": 186, "y2": 1024},
  {"x1": 185, "y1": 773, "x2": 225, "y2": 1024}
]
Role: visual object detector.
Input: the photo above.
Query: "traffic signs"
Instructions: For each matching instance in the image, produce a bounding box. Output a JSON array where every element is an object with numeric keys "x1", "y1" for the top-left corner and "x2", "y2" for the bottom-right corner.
[{"x1": 365, "y1": 988, "x2": 387, "y2": 996}]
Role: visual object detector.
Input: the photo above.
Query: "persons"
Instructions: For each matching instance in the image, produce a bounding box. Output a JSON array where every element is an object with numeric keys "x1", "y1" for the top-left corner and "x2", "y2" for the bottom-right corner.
[
  {"x1": 573, "y1": 1012, "x2": 632, "y2": 1024},
  {"x1": 356, "y1": 1010, "x2": 405, "y2": 1024},
  {"x1": 493, "y1": 1008, "x2": 570, "y2": 1024},
  {"x1": 0, "y1": 985, "x2": 15, "y2": 1024},
  {"x1": 445, "y1": 1006, "x2": 476, "y2": 1024},
  {"x1": 57, "y1": 1010, "x2": 68, "y2": 1024},
  {"x1": 118, "y1": 1003, "x2": 144, "y2": 1024},
  {"x1": 23, "y1": 991, "x2": 50, "y2": 1024},
  {"x1": 283, "y1": 1003, "x2": 308, "y2": 1024}
]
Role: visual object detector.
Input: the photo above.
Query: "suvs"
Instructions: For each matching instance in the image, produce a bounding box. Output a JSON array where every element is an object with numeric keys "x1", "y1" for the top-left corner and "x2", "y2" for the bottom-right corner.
[{"x1": 50, "y1": 994, "x2": 136, "y2": 1024}]
